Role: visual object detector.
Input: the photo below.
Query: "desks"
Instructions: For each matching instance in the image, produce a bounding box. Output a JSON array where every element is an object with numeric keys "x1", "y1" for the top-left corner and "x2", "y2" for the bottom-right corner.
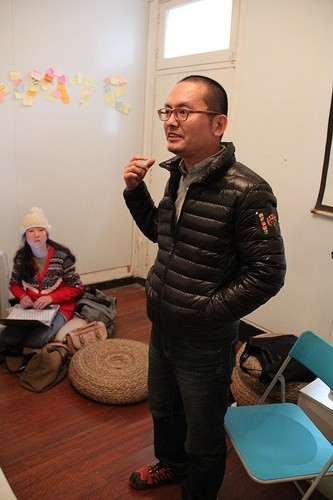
[{"x1": 296, "y1": 378, "x2": 333, "y2": 499}]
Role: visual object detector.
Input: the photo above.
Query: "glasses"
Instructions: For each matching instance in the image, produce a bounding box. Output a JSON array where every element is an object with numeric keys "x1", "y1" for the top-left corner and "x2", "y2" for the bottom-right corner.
[{"x1": 157, "y1": 107, "x2": 221, "y2": 122}]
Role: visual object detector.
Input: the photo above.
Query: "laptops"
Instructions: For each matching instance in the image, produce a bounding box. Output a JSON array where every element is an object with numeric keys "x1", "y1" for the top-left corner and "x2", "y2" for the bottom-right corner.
[{"x1": 0, "y1": 304, "x2": 61, "y2": 330}]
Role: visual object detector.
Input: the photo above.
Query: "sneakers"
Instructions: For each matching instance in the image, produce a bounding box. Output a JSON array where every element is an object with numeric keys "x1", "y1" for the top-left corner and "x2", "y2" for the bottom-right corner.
[{"x1": 129, "y1": 462, "x2": 186, "y2": 491}]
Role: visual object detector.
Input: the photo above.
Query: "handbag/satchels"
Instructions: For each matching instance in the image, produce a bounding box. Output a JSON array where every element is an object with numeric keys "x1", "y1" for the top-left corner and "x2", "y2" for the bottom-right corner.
[
  {"x1": 64, "y1": 319, "x2": 108, "y2": 361},
  {"x1": 17, "y1": 345, "x2": 71, "y2": 393},
  {"x1": 236, "y1": 333, "x2": 321, "y2": 387},
  {"x1": 73, "y1": 286, "x2": 118, "y2": 338}
]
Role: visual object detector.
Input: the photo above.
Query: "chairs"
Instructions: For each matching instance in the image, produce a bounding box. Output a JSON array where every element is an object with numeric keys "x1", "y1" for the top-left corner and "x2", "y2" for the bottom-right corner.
[{"x1": 224, "y1": 330, "x2": 333, "y2": 500}]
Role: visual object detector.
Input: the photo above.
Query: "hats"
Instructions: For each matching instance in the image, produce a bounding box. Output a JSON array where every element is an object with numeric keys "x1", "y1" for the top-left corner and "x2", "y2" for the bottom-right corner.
[{"x1": 19, "y1": 206, "x2": 51, "y2": 237}]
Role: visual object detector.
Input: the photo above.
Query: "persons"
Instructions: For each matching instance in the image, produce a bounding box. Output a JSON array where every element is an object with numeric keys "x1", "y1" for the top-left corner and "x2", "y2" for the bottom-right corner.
[
  {"x1": 123, "y1": 75, "x2": 286, "y2": 500},
  {"x1": 0, "y1": 206, "x2": 84, "y2": 349}
]
